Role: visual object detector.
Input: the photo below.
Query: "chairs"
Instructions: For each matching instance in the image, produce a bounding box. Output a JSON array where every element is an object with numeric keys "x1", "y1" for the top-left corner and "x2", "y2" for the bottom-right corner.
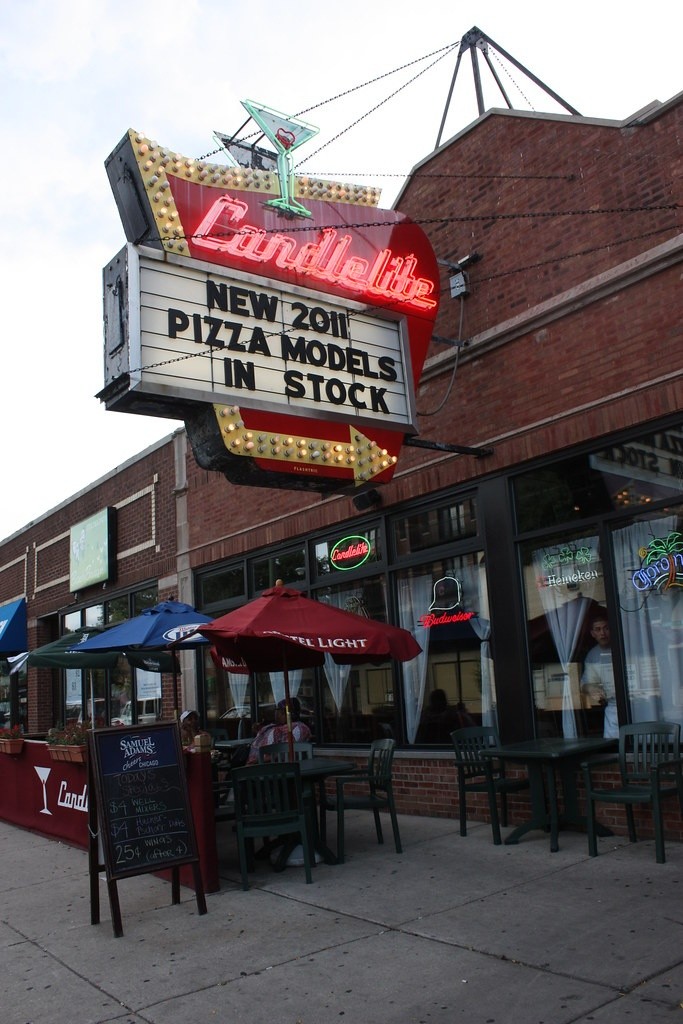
[
  {"x1": 318, "y1": 739, "x2": 403, "y2": 862},
  {"x1": 231, "y1": 761, "x2": 317, "y2": 891},
  {"x1": 211, "y1": 765, "x2": 235, "y2": 822},
  {"x1": 258, "y1": 742, "x2": 314, "y2": 762},
  {"x1": 580, "y1": 721, "x2": 683, "y2": 864},
  {"x1": 450, "y1": 727, "x2": 530, "y2": 846}
]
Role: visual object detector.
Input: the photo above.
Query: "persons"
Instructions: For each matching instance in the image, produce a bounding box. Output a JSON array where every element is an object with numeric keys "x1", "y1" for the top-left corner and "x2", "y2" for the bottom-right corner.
[
  {"x1": 248, "y1": 697, "x2": 310, "y2": 766},
  {"x1": 179, "y1": 710, "x2": 201, "y2": 746},
  {"x1": 428, "y1": 689, "x2": 478, "y2": 740},
  {"x1": 578, "y1": 615, "x2": 620, "y2": 741}
]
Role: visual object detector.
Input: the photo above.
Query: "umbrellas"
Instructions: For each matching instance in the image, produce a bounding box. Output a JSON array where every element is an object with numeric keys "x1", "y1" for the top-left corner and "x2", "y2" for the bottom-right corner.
[
  {"x1": 6, "y1": 626, "x2": 183, "y2": 730},
  {"x1": 63, "y1": 593, "x2": 217, "y2": 722},
  {"x1": 195, "y1": 582, "x2": 426, "y2": 766}
]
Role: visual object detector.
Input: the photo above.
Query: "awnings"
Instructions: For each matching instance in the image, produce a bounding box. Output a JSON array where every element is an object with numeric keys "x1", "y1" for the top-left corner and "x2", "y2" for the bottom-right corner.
[{"x1": 0, "y1": 598, "x2": 28, "y2": 652}]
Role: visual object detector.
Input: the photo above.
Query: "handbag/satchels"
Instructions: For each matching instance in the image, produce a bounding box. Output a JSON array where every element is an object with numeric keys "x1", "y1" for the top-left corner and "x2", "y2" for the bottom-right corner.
[{"x1": 231, "y1": 744, "x2": 251, "y2": 766}]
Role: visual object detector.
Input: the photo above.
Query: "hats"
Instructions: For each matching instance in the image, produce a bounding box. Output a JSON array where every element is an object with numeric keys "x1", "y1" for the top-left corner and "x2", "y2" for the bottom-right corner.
[
  {"x1": 180, "y1": 710, "x2": 199, "y2": 723},
  {"x1": 274, "y1": 698, "x2": 300, "y2": 710}
]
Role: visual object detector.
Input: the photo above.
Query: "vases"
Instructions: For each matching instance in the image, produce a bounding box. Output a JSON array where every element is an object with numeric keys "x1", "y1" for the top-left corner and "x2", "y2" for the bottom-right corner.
[
  {"x1": 46, "y1": 742, "x2": 87, "y2": 762},
  {"x1": 0, "y1": 739, "x2": 24, "y2": 753}
]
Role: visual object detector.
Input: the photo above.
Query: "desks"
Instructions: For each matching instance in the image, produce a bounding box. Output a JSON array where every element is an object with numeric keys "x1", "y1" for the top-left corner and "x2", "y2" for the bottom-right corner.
[
  {"x1": 239, "y1": 759, "x2": 354, "y2": 864},
  {"x1": 215, "y1": 739, "x2": 254, "y2": 762},
  {"x1": 478, "y1": 737, "x2": 618, "y2": 856}
]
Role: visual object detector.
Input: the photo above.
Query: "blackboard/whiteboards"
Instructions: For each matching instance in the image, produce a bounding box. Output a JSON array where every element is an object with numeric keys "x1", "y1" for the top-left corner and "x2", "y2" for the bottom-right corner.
[{"x1": 88, "y1": 720, "x2": 200, "y2": 883}]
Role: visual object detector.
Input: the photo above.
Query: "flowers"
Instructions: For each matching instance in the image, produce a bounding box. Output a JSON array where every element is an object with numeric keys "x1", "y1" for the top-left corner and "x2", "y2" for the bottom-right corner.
[
  {"x1": 46, "y1": 721, "x2": 93, "y2": 746},
  {"x1": 0, "y1": 725, "x2": 23, "y2": 739}
]
